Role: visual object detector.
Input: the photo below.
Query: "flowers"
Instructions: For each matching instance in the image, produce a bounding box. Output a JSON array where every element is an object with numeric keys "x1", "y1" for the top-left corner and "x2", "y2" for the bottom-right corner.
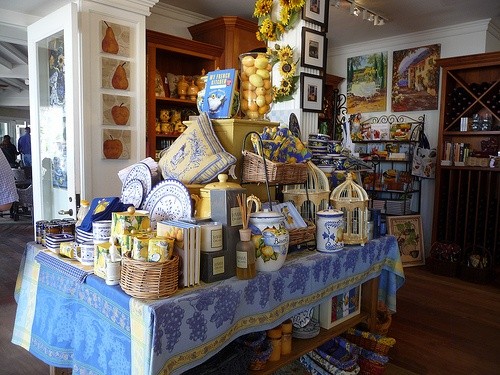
[{"x1": 252, "y1": 0, "x2": 305, "y2": 103}]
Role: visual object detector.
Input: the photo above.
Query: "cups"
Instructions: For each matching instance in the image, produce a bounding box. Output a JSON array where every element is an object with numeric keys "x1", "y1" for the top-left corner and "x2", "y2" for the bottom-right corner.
[
  {"x1": 154, "y1": 110, "x2": 186, "y2": 134},
  {"x1": 316, "y1": 206, "x2": 345, "y2": 253},
  {"x1": 238, "y1": 52, "x2": 274, "y2": 121},
  {"x1": 92, "y1": 220, "x2": 112, "y2": 243},
  {"x1": 73, "y1": 242, "x2": 94, "y2": 266}
]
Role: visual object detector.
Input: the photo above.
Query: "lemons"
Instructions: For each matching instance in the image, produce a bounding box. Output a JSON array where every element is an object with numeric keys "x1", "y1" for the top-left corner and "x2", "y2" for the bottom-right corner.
[{"x1": 240, "y1": 55, "x2": 272, "y2": 119}]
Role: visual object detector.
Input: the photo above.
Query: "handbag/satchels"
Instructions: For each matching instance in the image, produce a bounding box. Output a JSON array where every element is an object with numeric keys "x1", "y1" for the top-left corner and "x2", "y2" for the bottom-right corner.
[{"x1": 411, "y1": 133, "x2": 437, "y2": 179}]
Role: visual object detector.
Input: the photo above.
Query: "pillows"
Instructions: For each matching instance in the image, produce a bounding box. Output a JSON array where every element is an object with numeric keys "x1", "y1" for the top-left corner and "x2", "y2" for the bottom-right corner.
[{"x1": 159, "y1": 111, "x2": 237, "y2": 185}]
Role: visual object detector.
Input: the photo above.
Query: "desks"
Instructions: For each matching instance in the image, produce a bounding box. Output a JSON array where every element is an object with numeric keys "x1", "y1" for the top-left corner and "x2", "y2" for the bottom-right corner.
[{"x1": 11, "y1": 234, "x2": 405, "y2": 375}]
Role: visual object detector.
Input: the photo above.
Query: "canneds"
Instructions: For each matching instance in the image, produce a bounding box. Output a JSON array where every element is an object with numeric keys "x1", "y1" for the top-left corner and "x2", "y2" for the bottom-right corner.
[{"x1": 36, "y1": 218, "x2": 76, "y2": 247}]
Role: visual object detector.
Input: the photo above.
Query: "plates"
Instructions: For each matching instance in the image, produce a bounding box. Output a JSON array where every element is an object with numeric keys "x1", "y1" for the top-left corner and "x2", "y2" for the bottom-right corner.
[
  {"x1": 141, "y1": 178, "x2": 192, "y2": 232},
  {"x1": 121, "y1": 179, "x2": 143, "y2": 210},
  {"x1": 121, "y1": 163, "x2": 152, "y2": 200}
]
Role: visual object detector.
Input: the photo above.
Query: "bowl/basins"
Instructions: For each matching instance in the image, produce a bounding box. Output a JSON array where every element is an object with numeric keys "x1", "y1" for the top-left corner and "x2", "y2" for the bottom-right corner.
[{"x1": 307, "y1": 133, "x2": 346, "y2": 172}]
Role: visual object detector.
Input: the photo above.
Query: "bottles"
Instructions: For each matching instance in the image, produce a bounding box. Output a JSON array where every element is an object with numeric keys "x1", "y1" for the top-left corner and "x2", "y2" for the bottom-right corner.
[
  {"x1": 249, "y1": 208, "x2": 289, "y2": 272},
  {"x1": 267, "y1": 319, "x2": 292, "y2": 362},
  {"x1": 177, "y1": 69, "x2": 208, "y2": 114},
  {"x1": 471, "y1": 120, "x2": 481, "y2": 131},
  {"x1": 236, "y1": 229, "x2": 256, "y2": 281},
  {"x1": 104, "y1": 245, "x2": 122, "y2": 285},
  {"x1": 481, "y1": 119, "x2": 491, "y2": 130}
]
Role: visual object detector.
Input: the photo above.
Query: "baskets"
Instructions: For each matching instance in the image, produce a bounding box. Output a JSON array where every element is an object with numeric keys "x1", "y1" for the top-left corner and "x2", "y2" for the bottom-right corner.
[
  {"x1": 120, "y1": 251, "x2": 179, "y2": 299},
  {"x1": 340, "y1": 327, "x2": 396, "y2": 356},
  {"x1": 242, "y1": 150, "x2": 308, "y2": 184},
  {"x1": 235, "y1": 330, "x2": 272, "y2": 371},
  {"x1": 269, "y1": 336, "x2": 389, "y2": 375},
  {"x1": 428, "y1": 240, "x2": 461, "y2": 278},
  {"x1": 461, "y1": 245, "x2": 492, "y2": 281},
  {"x1": 360, "y1": 304, "x2": 391, "y2": 336},
  {"x1": 368, "y1": 198, "x2": 386, "y2": 213},
  {"x1": 288, "y1": 219, "x2": 316, "y2": 246},
  {"x1": 386, "y1": 200, "x2": 411, "y2": 215}
]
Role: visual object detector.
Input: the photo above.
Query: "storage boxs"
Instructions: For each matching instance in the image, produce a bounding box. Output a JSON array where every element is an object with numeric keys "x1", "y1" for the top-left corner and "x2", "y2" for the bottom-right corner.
[
  {"x1": 289, "y1": 219, "x2": 316, "y2": 246},
  {"x1": 373, "y1": 200, "x2": 410, "y2": 215},
  {"x1": 313, "y1": 284, "x2": 361, "y2": 329}
]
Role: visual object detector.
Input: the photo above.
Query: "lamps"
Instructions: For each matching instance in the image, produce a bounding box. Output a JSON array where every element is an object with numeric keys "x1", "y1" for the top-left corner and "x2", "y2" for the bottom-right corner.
[{"x1": 331, "y1": 0, "x2": 391, "y2": 26}]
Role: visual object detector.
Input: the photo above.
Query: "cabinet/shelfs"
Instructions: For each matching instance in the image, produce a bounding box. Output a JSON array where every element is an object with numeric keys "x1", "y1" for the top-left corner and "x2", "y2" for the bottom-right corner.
[
  {"x1": 352, "y1": 113, "x2": 425, "y2": 217},
  {"x1": 431, "y1": 51, "x2": 500, "y2": 284},
  {"x1": 146, "y1": 30, "x2": 224, "y2": 162}
]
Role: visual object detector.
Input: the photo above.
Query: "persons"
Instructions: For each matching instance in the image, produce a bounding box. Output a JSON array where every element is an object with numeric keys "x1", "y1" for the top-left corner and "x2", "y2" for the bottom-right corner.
[
  {"x1": 18, "y1": 127, "x2": 32, "y2": 178},
  {"x1": 0, "y1": 148, "x2": 19, "y2": 211},
  {"x1": 0, "y1": 135, "x2": 17, "y2": 168}
]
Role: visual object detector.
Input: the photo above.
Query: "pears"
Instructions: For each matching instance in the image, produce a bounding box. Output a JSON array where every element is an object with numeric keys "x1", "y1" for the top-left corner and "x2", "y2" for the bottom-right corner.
[
  {"x1": 112, "y1": 61, "x2": 128, "y2": 90},
  {"x1": 101, "y1": 21, "x2": 119, "y2": 55}
]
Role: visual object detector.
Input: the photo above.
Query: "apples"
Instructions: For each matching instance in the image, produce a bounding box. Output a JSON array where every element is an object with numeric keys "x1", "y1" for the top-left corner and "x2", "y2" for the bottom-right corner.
[
  {"x1": 103, "y1": 135, "x2": 123, "y2": 159},
  {"x1": 111, "y1": 103, "x2": 130, "y2": 125}
]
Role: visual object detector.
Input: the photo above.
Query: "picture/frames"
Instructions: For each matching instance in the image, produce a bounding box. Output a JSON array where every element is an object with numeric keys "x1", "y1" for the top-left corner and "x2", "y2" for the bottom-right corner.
[
  {"x1": 301, "y1": 27, "x2": 326, "y2": 71},
  {"x1": 301, "y1": 0, "x2": 326, "y2": 26},
  {"x1": 300, "y1": 72, "x2": 324, "y2": 113},
  {"x1": 386, "y1": 215, "x2": 425, "y2": 268}
]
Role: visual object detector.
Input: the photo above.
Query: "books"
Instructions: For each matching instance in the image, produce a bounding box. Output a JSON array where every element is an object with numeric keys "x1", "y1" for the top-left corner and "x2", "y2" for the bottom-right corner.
[
  {"x1": 443, "y1": 141, "x2": 472, "y2": 165},
  {"x1": 461, "y1": 118, "x2": 468, "y2": 131}
]
